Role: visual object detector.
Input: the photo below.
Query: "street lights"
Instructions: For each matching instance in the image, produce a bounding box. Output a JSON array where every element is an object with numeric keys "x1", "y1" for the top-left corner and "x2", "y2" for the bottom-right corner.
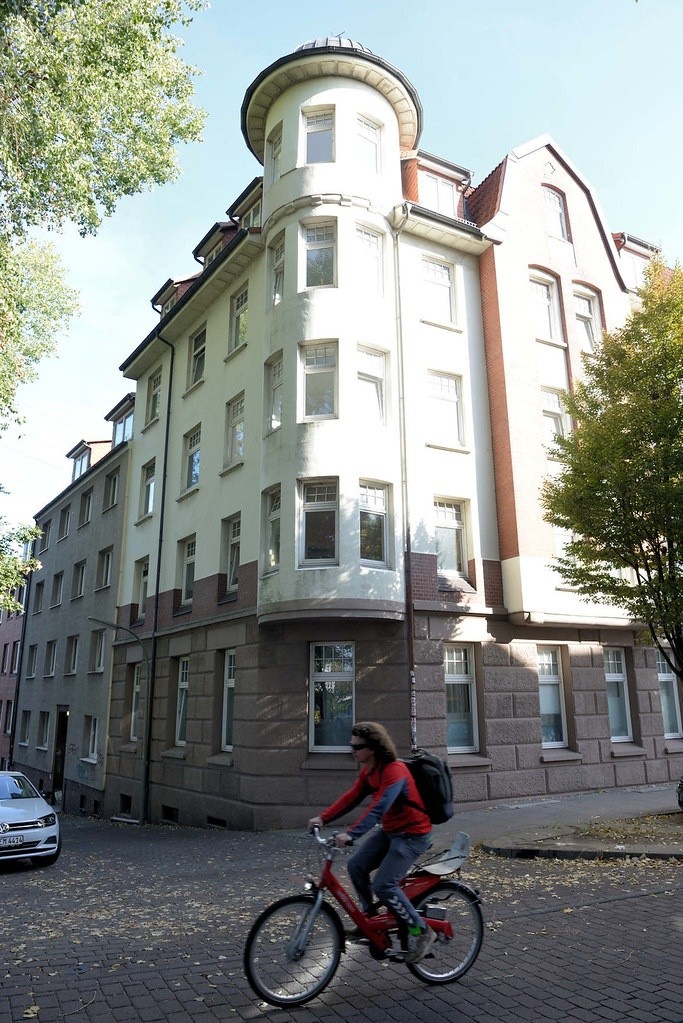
[{"x1": 88, "y1": 616, "x2": 150, "y2": 827}]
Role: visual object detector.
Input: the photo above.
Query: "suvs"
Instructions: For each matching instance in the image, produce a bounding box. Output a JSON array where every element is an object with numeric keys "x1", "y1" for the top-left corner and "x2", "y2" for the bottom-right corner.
[{"x1": 0, "y1": 771, "x2": 62, "y2": 867}]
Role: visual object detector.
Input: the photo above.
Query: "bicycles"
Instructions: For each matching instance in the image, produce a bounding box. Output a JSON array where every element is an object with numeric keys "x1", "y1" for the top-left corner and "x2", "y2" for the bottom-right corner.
[{"x1": 242, "y1": 823, "x2": 486, "y2": 1006}]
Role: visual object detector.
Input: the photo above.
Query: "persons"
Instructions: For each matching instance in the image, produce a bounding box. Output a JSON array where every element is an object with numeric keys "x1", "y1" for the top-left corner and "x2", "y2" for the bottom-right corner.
[{"x1": 308, "y1": 721, "x2": 438, "y2": 963}]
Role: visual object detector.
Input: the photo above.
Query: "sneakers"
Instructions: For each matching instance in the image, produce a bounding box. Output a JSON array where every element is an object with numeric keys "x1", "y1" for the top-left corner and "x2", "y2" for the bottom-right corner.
[
  {"x1": 406, "y1": 924, "x2": 437, "y2": 963},
  {"x1": 346, "y1": 914, "x2": 380, "y2": 939}
]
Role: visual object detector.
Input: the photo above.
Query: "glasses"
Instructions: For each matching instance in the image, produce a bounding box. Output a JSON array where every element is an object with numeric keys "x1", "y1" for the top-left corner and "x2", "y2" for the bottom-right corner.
[{"x1": 349, "y1": 741, "x2": 369, "y2": 750}]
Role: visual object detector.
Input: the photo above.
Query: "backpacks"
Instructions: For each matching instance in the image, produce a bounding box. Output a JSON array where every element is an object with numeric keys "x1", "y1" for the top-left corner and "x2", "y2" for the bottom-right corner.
[{"x1": 379, "y1": 748, "x2": 455, "y2": 824}]
